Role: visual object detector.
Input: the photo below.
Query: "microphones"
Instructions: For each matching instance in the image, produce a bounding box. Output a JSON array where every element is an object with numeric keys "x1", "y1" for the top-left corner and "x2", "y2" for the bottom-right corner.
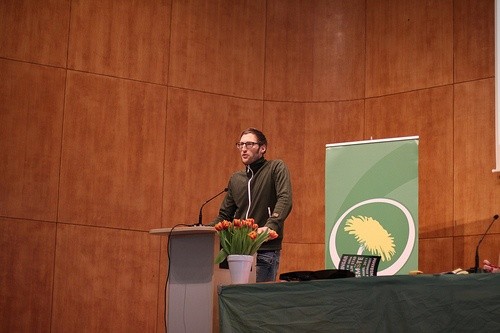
[
  {"x1": 194, "y1": 188, "x2": 229, "y2": 226},
  {"x1": 466, "y1": 215, "x2": 499, "y2": 273}
]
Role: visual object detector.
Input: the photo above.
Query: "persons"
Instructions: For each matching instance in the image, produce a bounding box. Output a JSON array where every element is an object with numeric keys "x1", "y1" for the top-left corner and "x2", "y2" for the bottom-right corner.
[{"x1": 209, "y1": 127, "x2": 292, "y2": 282}]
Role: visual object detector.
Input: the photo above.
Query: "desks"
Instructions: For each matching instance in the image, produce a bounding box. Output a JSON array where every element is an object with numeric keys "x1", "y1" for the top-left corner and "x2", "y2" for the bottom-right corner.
[{"x1": 217, "y1": 272, "x2": 500, "y2": 333}]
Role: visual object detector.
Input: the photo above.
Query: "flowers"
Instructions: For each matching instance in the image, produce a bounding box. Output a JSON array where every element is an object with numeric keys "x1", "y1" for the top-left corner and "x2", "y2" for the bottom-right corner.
[{"x1": 214, "y1": 218, "x2": 278, "y2": 265}]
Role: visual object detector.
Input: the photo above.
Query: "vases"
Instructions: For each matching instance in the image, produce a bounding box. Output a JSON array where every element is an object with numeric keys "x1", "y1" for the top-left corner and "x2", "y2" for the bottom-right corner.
[{"x1": 227, "y1": 255, "x2": 254, "y2": 284}]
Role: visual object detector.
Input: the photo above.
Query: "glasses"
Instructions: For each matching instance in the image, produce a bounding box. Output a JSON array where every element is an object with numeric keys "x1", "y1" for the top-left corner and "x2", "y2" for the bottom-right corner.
[{"x1": 237, "y1": 141, "x2": 261, "y2": 149}]
